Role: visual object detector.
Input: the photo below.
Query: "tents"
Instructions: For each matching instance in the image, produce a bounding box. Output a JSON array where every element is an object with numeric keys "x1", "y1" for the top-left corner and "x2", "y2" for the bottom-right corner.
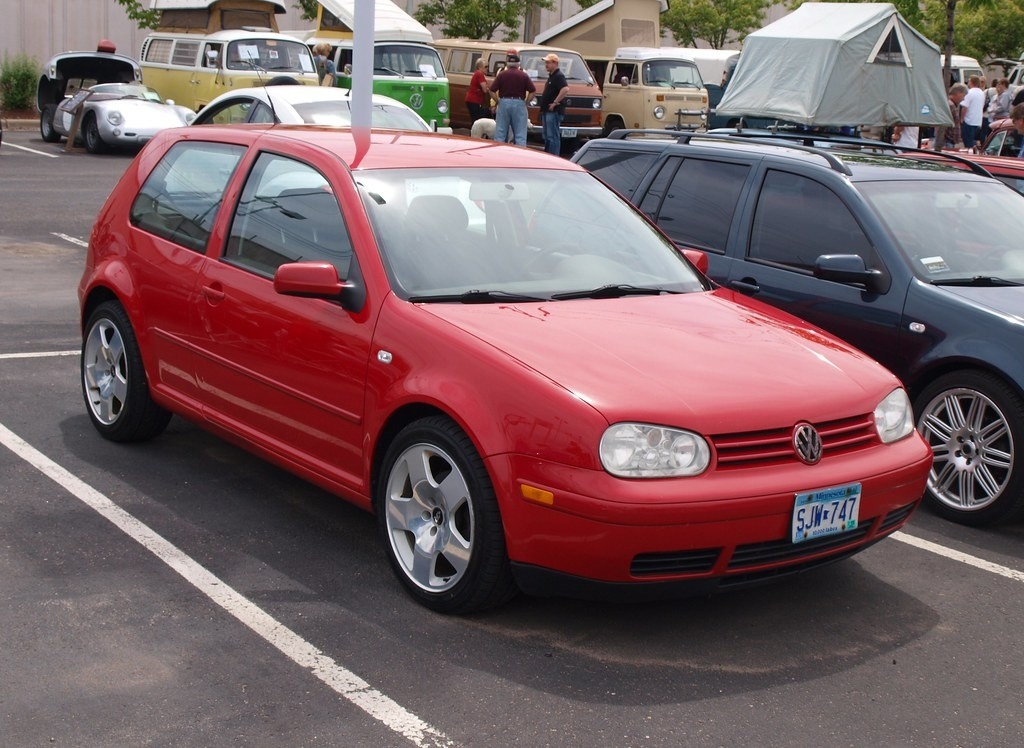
[{"x1": 717, "y1": 1, "x2": 954, "y2": 126}]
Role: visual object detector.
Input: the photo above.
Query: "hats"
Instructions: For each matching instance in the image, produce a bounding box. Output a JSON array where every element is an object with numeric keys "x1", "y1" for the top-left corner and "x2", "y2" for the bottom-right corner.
[
  {"x1": 542, "y1": 54, "x2": 559, "y2": 63},
  {"x1": 507, "y1": 55, "x2": 520, "y2": 62},
  {"x1": 506, "y1": 48, "x2": 517, "y2": 56}
]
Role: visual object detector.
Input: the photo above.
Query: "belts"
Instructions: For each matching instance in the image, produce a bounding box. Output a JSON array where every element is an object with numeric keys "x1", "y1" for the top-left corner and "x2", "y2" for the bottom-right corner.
[{"x1": 501, "y1": 96, "x2": 524, "y2": 100}]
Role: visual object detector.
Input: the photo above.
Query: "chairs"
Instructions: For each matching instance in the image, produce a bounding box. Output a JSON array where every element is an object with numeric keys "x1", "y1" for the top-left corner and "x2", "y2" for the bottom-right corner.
[
  {"x1": 257, "y1": 47, "x2": 278, "y2": 69},
  {"x1": 271, "y1": 189, "x2": 352, "y2": 279},
  {"x1": 229, "y1": 52, "x2": 255, "y2": 71},
  {"x1": 402, "y1": 196, "x2": 484, "y2": 288}
]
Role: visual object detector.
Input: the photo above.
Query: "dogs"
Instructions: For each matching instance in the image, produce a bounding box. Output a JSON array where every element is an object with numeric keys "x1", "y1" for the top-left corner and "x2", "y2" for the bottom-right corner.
[{"x1": 470, "y1": 118, "x2": 533, "y2": 145}]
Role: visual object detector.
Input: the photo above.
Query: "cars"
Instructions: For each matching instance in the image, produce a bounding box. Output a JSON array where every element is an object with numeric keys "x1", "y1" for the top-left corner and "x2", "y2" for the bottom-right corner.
[
  {"x1": 77, "y1": 122, "x2": 933, "y2": 615},
  {"x1": 166, "y1": 85, "x2": 486, "y2": 230},
  {"x1": 895, "y1": 151, "x2": 1024, "y2": 196}
]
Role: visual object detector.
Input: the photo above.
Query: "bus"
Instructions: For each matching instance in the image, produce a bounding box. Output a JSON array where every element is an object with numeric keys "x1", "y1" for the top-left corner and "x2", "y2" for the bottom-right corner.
[
  {"x1": 238, "y1": 0, "x2": 453, "y2": 135},
  {"x1": 523, "y1": 56, "x2": 709, "y2": 137},
  {"x1": 414, "y1": 39, "x2": 603, "y2": 135},
  {"x1": 139, "y1": 0, "x2": 319, "y2": 124}
]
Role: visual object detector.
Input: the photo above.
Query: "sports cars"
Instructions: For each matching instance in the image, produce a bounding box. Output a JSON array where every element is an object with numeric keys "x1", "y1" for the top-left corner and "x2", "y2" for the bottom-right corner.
[{"x1": 37, "y1": 39, "x2": 197, "y2": 154}]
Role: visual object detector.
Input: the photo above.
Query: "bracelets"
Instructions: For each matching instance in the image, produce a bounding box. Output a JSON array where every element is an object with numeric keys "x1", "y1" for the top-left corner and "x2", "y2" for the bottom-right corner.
[{"x1": 552, "y1": 99, "x2": 561, "y2": 105}]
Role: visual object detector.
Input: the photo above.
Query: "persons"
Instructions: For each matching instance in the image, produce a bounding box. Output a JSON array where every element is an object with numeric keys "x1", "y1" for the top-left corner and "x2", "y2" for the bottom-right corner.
[
  {"x1": 490, "y1": 46, "x2": 529, "y2": 145},
  {"x1": 796, "y1": 75, "x2": 1011, "y2": 153},
  {"x1": 959, "y1": 74, "x2": 984, "y2": 154},
  {"x1": 1010, "y1": 101, "x2": 1024, "y2": 194},
  {"x1": 931, "y1": 82, "x2": 968, "y2": 152},
  {"x1": 891, "y1": 125, "x2": 918, "y2": 154},
  {"x1": 539, "y1": 53, "x2": 569, "y2": 158},
  {"x1": 312, "y1": 42, "x2": 337, "y2": 87},
  {"x1": 489, "y1": 55, "x2": 536, "y2": 147},
  {"x1": 465, "y1": 57, "x2": 489, "y2": 123}
]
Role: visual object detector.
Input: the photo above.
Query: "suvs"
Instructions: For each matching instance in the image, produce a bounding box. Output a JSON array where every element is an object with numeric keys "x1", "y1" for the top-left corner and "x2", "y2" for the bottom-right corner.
[{"x1": 524, "y1": 129, "x2": 1024, "y2": 526}]
